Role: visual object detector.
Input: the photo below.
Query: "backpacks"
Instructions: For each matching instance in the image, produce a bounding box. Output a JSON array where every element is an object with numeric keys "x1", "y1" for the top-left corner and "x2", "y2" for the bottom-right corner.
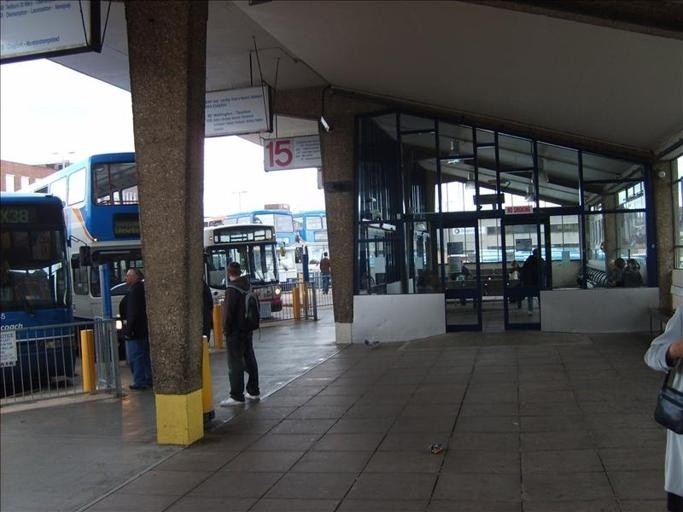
[{"x1": 226, "y1": 283, "x2": 261, "y2": 331}]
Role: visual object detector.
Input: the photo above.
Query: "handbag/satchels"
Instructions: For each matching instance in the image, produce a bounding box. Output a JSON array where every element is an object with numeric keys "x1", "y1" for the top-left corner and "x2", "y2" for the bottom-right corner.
[{"x1": 654, "y1": 369, "x2": 683, "y2": 436}]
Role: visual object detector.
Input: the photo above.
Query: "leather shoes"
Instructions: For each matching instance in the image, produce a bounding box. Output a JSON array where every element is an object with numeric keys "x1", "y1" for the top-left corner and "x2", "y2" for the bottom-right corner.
[{"x1": 129, "y1": 384, "x2": 146, "y2": 390}]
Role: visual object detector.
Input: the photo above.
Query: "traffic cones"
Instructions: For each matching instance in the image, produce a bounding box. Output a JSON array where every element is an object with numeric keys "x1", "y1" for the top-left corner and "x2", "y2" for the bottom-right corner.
[{"x1": 200, "y1": 334, "x2": 216, "y2": 420}]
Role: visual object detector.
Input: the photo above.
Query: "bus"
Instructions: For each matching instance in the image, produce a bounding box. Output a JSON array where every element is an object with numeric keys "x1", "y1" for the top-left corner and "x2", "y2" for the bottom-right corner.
[
  {"x1": 2, "y1": 192, "x2": 77, "y2": 385},
  {"x1": 480, "y1": 250, "x2": 648, "y2": 266},
  {"x1": 293, "y1": 210, "x2": 332, "y2": 289},
  {"x1": 203, "y1": 216, "x2": 283, "y2": 319},
  {"x1": 14, "y1": 152, "x2": 145, "y2": 361},
  {"x1": 222, "y1": 210, "x2": 299, "y2": 291}
]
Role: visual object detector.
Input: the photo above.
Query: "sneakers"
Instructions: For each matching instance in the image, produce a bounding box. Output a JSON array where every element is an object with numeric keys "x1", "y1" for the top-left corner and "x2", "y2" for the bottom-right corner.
[
  {"x1": 245, "y1": 393, "x2": 261, "y2": 400},
  {"x1": 219, "y1": 397, "x2": 245, "y2": 407}
]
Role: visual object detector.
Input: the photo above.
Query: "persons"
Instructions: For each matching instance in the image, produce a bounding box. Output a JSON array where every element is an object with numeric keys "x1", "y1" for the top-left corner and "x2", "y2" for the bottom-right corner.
[
  {"x1": 465, "y1": 271, "x2": 473, "y2": 280},
  {"x1": 118, "y1": 266, "x2": 153, "y2": 390},
  {"x1": 624, "y1": 259, "x2": 644, "y2": 285},
  {"x1": 320, "y1": 252, "x2": 331, "y2": 294},
  {"x1": 203, "y1": 280, "x2": 214, "y2": 342},
  {"x1": 450, "y1": 265, "x2": 469, "y2": 306},
  {"x1": 220, "y1": 260, "x2": 260, "y2": 406},
  {"x1": 612, "y1": 257, "x2": 626, "y2": 286},
  {"x1": 519, "y1": 248, "x2": 547, "y2": 315},
  {"x1": 643, "y1": 302, "x2": 683, "y2": 512},
  {"x1": 244, "y1": 247, "x2": 256, "y2": 275},
  {"x1": 508, "y1": 259, "x2": 525, "y2": 303}
]
